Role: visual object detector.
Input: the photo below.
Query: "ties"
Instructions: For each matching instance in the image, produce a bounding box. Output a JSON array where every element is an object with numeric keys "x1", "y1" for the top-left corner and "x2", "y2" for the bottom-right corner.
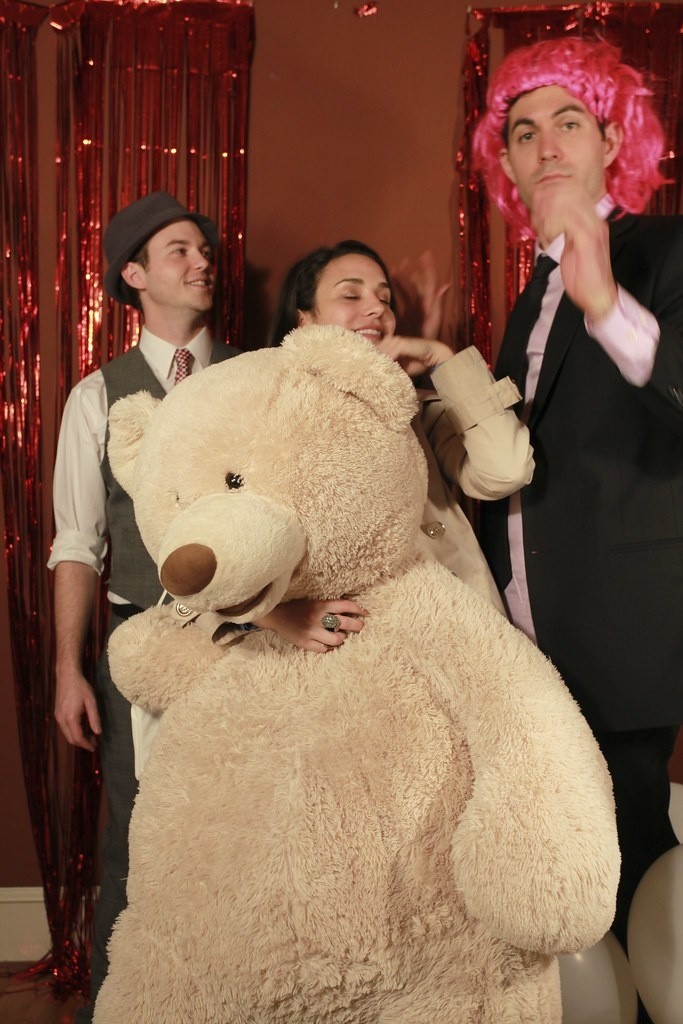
[
  {"x1": 479, "y1": 254, "x2": 559, "y2": 591},
  {"x1": 174, "y1": 348, "x2": 195, "y2": 386}
]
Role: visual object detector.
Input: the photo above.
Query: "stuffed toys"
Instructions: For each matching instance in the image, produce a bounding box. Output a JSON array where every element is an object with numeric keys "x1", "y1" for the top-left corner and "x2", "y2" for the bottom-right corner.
[{"x1": 92, "y1": 323, "x2": 619, "y2": 1022}]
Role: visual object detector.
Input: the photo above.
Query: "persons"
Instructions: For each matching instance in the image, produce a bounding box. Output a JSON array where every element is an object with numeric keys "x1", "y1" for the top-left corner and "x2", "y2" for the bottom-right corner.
[
  {"x1": 466, "y1": 33, "x2": 683, "y2": 1022},
  {"x1": 51, "y1": 195, "x2": 254, "y2": 1018},
  {"x1": 166, "y1": 230, "x2": 534, "y2": 628}
]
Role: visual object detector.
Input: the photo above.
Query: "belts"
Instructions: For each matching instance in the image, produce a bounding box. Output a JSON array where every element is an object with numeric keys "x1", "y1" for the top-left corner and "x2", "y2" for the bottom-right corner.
[{"x1": 112, "y1": 603, "x2": 147, "y2": 620}]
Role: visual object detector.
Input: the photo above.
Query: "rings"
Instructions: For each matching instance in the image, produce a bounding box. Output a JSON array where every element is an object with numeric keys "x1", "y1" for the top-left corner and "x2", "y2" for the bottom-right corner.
[{"x1": 320, "y1": 614, "x2": 340, "y2": 630}]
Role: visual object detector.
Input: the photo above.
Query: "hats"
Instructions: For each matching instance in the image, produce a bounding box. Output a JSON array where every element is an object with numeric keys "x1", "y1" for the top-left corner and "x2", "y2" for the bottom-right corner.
[{"x1": 105, "y1": 191, "x2": 217, "y2": 305}]
